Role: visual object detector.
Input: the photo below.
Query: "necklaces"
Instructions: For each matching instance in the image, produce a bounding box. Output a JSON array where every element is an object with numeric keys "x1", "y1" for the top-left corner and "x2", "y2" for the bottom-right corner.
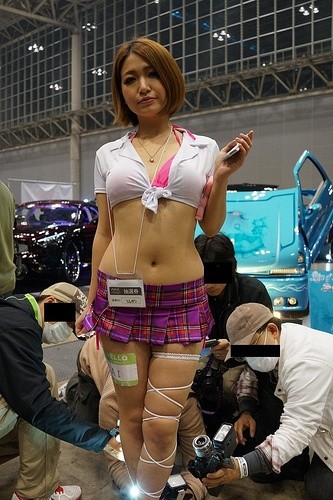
[{"x1": 136, "y1": 128, "x2": 170, "y2": 163}]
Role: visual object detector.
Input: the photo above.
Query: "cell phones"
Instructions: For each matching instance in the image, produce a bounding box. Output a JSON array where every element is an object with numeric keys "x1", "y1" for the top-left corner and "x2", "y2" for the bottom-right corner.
[
  {"x1": 205, "y1": 339, "x2": 219, "y2": 348},
  {"x1": 227, "y1": 143, "x2": 241, "y2": 157}
]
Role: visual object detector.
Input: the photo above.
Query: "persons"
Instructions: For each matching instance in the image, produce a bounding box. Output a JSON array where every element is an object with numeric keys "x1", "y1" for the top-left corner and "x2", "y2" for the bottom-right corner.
[
  {"x1": 0, "y1": 182, "x2": 17, "y2": 301},
  {"x1": 0, "y1": 233, "x2": 333, "y2": 500},
  {"x1": 73, "y1": 37, "x2": 254, "y2": 500}
]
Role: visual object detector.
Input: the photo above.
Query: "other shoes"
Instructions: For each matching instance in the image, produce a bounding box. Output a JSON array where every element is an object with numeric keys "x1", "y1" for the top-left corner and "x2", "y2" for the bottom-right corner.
[
  {"x1": 55, "y1": 382, "x2": 69, "y2": 405},
  {"x1": 251, "y1": 445, "x2": 311, "y2": 484}
]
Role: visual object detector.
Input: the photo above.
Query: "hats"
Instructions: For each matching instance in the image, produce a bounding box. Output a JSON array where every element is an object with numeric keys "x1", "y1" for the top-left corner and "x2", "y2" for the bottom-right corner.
[
  {"x1": 224, "y1": 303, "x2": 274, "y2": 363},
  {"x1": 40, "y1": 282, "x2": 89, "y2": 315}
]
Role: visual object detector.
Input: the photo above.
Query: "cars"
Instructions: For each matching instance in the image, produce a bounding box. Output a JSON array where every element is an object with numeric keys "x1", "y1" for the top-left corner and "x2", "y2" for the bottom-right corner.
[{"x1": 13, "y1": 199, "x2": 98, "y2": 288}]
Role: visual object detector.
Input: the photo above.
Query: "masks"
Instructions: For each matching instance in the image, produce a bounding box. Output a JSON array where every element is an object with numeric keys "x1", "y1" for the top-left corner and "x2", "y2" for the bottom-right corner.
[
  {"x1": 43, "y1": 321, "x2": 72, "y2": 345},
  {"x1": 240, "y1": 331, "x2": 279, "y2": 372}
]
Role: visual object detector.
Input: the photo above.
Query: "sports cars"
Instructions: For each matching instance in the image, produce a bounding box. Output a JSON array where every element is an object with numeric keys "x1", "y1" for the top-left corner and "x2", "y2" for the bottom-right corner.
[{"x1": 193, "y1": 148, "x2": 333, "y2": 333}]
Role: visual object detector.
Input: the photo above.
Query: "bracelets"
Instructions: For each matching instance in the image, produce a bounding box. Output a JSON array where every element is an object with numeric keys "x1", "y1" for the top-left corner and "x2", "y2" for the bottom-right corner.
[{"x1": 234, "y1": 456, "x2": 249, "y2": 480}]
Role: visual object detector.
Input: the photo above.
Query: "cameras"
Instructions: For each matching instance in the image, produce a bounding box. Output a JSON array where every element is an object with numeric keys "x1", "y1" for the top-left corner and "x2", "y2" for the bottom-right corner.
[{"x1": 187, "y1": 422, "x2": 238, "y2": 497}]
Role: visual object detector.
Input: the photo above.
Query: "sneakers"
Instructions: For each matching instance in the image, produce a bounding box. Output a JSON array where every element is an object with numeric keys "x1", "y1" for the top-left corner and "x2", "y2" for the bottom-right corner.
[{"x1": 12, "y1": 485, "x2": 82, "y2": 500}]
